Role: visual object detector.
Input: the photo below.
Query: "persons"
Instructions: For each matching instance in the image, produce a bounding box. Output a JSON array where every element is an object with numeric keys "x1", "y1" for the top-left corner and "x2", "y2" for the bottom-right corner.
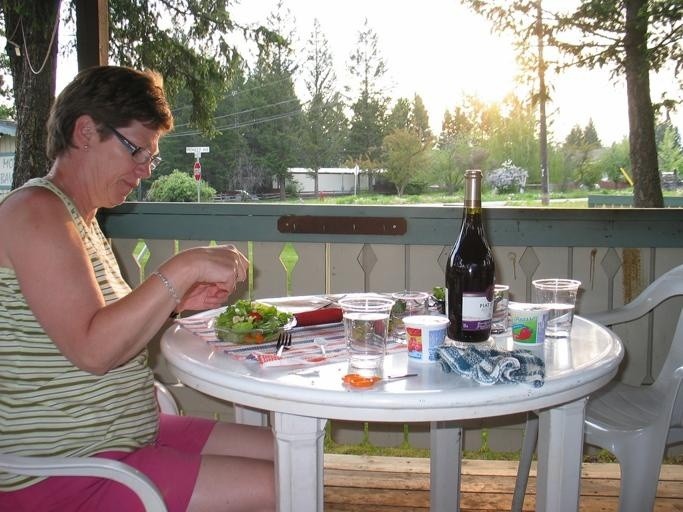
[{"x1": 0, "y1": 64, "x2": 277, "y2": 512}]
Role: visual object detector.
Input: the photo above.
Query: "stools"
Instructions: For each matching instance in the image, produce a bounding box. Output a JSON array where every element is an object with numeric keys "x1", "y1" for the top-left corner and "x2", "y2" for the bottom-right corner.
[{"x1": 2, "y1": 374, "x2": 181, "y2": 512}]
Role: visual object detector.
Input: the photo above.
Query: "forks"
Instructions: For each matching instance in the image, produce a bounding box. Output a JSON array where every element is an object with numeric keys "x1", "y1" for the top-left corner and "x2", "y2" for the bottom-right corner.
[{"x1": 272, "y1": 332, "x2": 293, "y2": 359}]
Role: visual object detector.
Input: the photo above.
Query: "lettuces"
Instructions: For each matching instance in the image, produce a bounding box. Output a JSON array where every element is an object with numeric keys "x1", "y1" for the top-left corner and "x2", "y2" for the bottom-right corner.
[{"x1": 214, "y1": 298, "x2": 292, "y2": 343}]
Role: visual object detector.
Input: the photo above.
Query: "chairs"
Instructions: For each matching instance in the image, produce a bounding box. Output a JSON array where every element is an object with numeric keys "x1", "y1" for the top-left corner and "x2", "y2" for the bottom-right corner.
[{"x1": 510, "y1": 263, "x2": 683, "y2": 512}]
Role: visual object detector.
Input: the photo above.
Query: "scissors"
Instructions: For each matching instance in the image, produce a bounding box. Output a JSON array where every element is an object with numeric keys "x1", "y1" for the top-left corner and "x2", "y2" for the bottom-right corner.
[{"x1": 343, "y1": 373, "x2": 418, "y2": 387}]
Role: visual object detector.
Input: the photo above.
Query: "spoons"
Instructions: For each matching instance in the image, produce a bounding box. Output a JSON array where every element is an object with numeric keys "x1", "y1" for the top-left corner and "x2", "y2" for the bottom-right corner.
[{"x1": 312, "y1": 336, "x2": 330, "y2": 359}]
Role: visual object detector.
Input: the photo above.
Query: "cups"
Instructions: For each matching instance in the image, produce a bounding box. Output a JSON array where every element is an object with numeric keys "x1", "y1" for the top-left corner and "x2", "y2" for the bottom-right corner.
[
  {"x1": 531, "y1": 277, "x2": 582, "y2": 340},
  {"x1": 509, "y1": 302, "x2": 550, "y2": 347},
  {"x1": 390, "y1": 291, "x2": 430, "y2": 346},
  {"x1": 490, "y1": 284, "x2": 513, "y2": 335},
  {"x1": 401, "y1": 314, "x2": 453, "y2": 364},
  {"x1": 336, "y1": 297, "x2": 396, "y2": 370}
]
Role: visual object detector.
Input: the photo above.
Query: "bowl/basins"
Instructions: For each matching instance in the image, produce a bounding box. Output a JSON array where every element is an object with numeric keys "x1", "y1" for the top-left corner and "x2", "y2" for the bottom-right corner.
[{"x1": 207, "y1": 314, "x2": 298, "y2": 344}]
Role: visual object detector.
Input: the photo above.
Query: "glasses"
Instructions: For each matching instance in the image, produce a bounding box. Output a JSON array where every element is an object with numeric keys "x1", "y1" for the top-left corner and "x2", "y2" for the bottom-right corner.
[{"x1": 111, "y1": 127, "x2": 161, "y2": 170}]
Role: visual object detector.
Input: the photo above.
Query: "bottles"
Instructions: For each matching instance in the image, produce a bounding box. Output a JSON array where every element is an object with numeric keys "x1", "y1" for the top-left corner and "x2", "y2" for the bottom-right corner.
[{"x1": 444, "y1": 169, "x2": 497, "y2": 343}]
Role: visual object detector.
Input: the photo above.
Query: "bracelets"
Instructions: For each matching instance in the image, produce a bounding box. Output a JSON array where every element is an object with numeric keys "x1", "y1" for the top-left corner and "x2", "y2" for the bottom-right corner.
[{"x1": 150, "y1": 270, "x2": 180, "y2": 304}]
